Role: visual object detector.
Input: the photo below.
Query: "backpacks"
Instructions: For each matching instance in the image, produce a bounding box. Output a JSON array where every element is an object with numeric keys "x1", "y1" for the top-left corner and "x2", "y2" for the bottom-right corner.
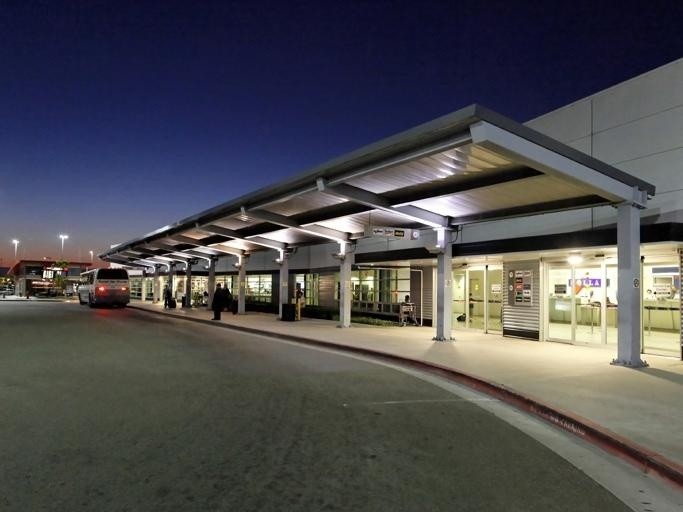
[{"x1": 166, "y1": 289, "x2": 172, "y2": 298}]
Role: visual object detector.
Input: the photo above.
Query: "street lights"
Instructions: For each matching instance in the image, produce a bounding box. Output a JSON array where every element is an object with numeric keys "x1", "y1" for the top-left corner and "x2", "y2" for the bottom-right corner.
[
  {"x1": 59, "y1": 235, "x2": 68, "y2": 256},
  {"x1": 13, "y1": 240, "x2": 20, "y2": 256}
]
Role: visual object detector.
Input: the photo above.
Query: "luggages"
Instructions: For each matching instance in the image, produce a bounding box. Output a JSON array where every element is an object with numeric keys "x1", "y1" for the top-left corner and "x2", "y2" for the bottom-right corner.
[{"x1": 168, "y1": 299, "x2": 177, "y2": 308}]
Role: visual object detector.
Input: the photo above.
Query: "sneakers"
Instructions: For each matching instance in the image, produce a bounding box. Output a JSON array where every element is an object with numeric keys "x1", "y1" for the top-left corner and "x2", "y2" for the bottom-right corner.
[{"x1": 211, "y1": 318, "x2": 221, "y2": 320}]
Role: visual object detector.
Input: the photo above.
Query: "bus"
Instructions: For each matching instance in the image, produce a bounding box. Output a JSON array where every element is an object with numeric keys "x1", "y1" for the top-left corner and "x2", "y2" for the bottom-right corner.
[{"x1": 76, "y1": 267, "x2": 131, "y2": 308}]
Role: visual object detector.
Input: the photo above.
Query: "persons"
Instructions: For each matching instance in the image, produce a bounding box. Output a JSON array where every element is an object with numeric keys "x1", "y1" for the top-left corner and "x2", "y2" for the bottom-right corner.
[
  {"x1": 222, "y1": 284, "x2": 230, "y2": 312},
  {"x1": 296, "y1": 287, "x2": 304, "y2": 318},
  {"x1": 211, "y1": 283, "x2": 224, "y2": 320},
  {"x1": 469, "y1": 293, "x2": 474, "y2": 320},
  {"x1": 401, "y1": 295, "x2": 410, "y2": 323},
  {"x1": 162, "y1": 284, "x2": 171, "y2": 309}
]
